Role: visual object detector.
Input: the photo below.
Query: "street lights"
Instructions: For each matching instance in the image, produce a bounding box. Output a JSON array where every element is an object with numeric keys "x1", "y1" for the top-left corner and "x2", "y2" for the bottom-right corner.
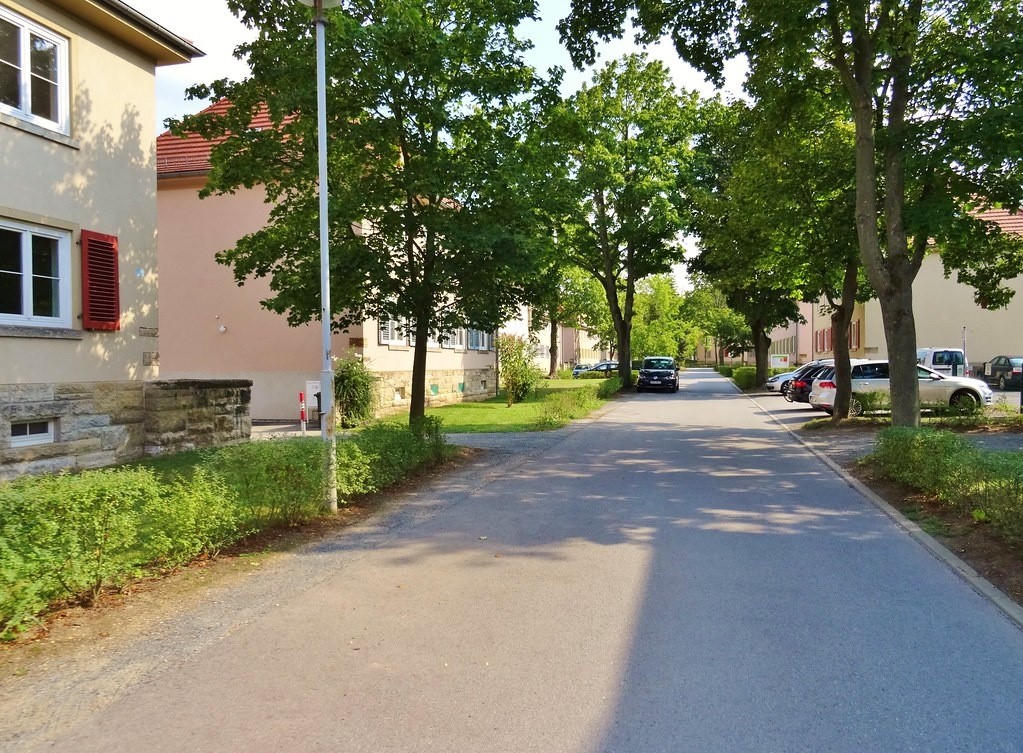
[{"x1": 299, "y1": 0, "x2": 345, "y2": 518}]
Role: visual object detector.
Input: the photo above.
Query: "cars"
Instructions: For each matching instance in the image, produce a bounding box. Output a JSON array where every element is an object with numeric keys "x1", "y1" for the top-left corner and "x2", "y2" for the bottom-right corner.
[
  {"x1": 977, "y1": 355, "x2": 1023, "y2": 391},
  {"x1": 766, "y1": 360, "x2": 872, "y2": 403},
  {"x1": 584, "y1": 361, "x2": 620, "y2": 371},
  {"x1": 572, "y1": 364, "x2": 593, "y2": 379},
  {"x1": 808, "y1": 359, "x2": 994, "y2": 416},
  {"x1": 637, "y1": 356, "x2": 681, "y2": 393}
]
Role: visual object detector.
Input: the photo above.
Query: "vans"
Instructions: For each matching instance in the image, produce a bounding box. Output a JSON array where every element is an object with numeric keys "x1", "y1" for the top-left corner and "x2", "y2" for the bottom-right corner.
[{"x1": 916, "y1": 347, "x2": 969, "y2": 377}]
